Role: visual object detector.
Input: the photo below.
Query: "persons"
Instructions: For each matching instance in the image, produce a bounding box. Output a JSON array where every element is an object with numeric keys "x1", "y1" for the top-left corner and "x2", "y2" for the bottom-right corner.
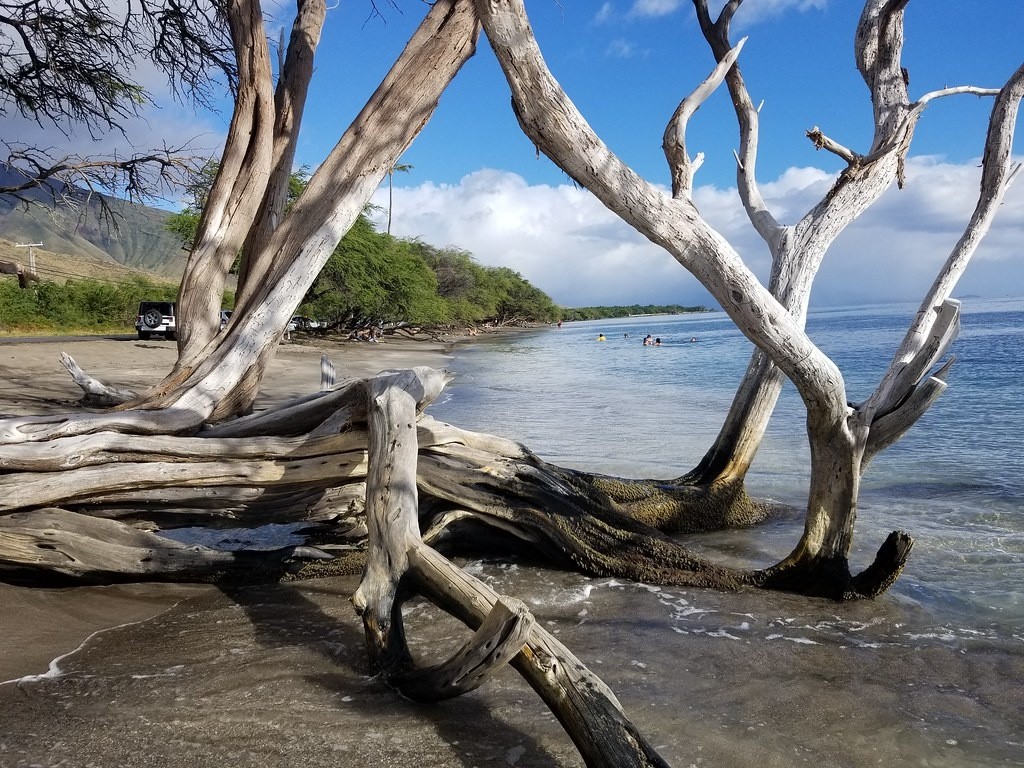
[
  {"x1": 345, "y1": 327, "x2": 384, "y2": 342},
  {"x1": 596, "y1": 334, "x2": 606, "y2": 341},
  {"x1": 556, "y1": 320, "x2": 563, "y2": 329},
  {"x1": 623, "y1": 334, "x2": 631, "y2": 338},
  {"x1": 691, "y1": 337, "x2": 697, "y2": 342},
  {"x1": 643, "y1": 334, "x2": 662, "y2": 347}
]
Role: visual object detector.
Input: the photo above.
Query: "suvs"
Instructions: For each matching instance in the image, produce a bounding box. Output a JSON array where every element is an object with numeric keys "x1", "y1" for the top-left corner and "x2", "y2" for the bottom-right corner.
[{"x1": 134, "y1": 300, "x2": 177, "y2": 340}]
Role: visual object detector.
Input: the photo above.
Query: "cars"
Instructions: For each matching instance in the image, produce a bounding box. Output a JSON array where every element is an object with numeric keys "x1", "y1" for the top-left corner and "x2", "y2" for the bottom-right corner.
[
  {"x1": 220, "y1": 309, "x2": 232, "y2": 331},
  {"x1": 285, "y1": 315, "x2": 327, "y2": 332}
]
